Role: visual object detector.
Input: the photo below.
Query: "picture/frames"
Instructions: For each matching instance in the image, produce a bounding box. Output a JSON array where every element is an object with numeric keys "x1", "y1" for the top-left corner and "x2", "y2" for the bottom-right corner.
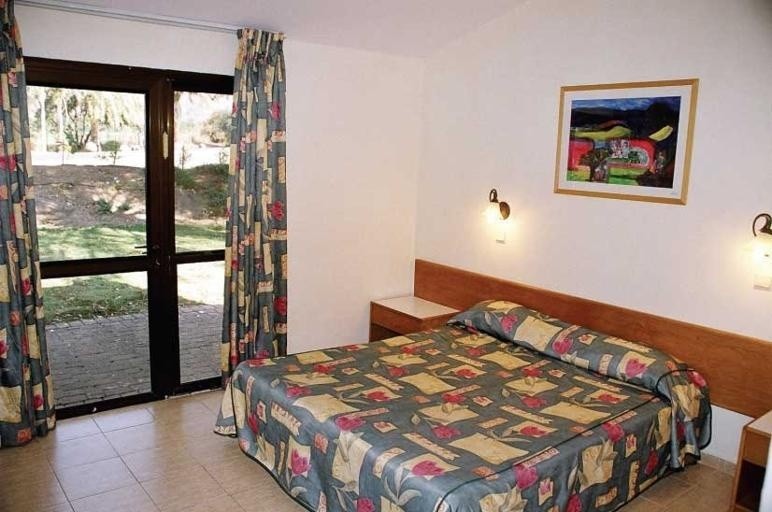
[{"x1": 553, "y1": 78, "x2": 699, "y2": 205}]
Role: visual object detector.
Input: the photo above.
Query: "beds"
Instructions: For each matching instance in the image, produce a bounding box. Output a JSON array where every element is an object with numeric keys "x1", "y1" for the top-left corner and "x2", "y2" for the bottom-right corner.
[{"x1": 213, "y1": 300, "x2": 711, "y2": 512}]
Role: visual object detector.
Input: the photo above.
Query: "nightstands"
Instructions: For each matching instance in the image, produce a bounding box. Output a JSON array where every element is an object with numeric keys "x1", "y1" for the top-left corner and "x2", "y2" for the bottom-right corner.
[
  {"x1": 729, "y1": 410, "x2": 772, "y2": 512},
  {"x1": 369, "y1": 295, "x2": 460, "y2": 343}
]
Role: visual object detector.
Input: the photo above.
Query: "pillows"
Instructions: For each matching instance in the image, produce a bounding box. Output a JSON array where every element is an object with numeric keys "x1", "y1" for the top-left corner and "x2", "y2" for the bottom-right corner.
[{"x1": 447, "y1": 299, "x2": 710, "y2": 469}]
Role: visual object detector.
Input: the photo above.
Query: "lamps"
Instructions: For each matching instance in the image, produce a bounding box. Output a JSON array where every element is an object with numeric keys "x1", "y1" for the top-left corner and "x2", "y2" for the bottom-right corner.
[
  {"x1": 482, "y1": 188, "x2": 510, "y2": 221},
  {"x1": 745, "y1": 214, "x2": 772, "y2": 258}
]
